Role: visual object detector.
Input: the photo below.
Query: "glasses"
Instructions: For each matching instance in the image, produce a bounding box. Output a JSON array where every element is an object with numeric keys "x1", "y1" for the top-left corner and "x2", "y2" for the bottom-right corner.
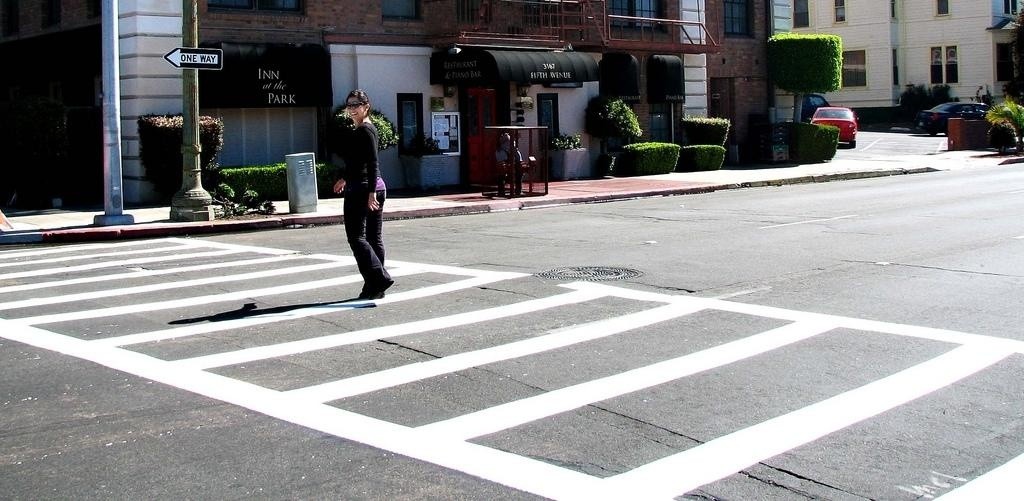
[{"x1": 347, "y1": 103, "x2": 364, "y2": 109}]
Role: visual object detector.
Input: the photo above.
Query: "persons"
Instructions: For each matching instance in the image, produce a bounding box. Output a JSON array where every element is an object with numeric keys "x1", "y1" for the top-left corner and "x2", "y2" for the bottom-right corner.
[
  {"x1": 494, "y1": 131, "x2": 530, "y2": 199},
  {"x1": 332, "y1": 88, "x2": 394, "y2": 302}
]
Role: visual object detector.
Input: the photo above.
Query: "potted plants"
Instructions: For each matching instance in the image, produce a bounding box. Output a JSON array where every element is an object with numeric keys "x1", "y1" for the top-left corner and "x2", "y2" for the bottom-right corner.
[
  {"x1": 543, "y1": 133, "x2": 591, "y2": 180},
  {"x1": 398, "y1": 133, "x2": 449, "y2": 187}
]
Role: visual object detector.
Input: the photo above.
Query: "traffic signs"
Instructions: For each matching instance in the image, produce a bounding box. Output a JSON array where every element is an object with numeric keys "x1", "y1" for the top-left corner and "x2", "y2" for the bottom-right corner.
[{"x1": 163, "y1": 47, "x2": 224, "y2": 70}]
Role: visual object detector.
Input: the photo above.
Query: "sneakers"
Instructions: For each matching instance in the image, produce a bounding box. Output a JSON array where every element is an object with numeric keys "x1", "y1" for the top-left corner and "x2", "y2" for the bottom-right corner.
[{"x1": 359, "y1": 278, "x2": 394, "y2": 299}]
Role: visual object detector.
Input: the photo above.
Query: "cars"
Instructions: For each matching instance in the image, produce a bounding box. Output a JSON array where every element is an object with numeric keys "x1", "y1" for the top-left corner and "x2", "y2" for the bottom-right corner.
[
  {"x1": 802, "y1": 94, "x2": 830, "y2": 121},
  {"x1": 807, "y1": 106, "x2": 857, "y2": 147},
  {"x1": 914, "y1": 102, "x2": 991, "y2": 136}
]
